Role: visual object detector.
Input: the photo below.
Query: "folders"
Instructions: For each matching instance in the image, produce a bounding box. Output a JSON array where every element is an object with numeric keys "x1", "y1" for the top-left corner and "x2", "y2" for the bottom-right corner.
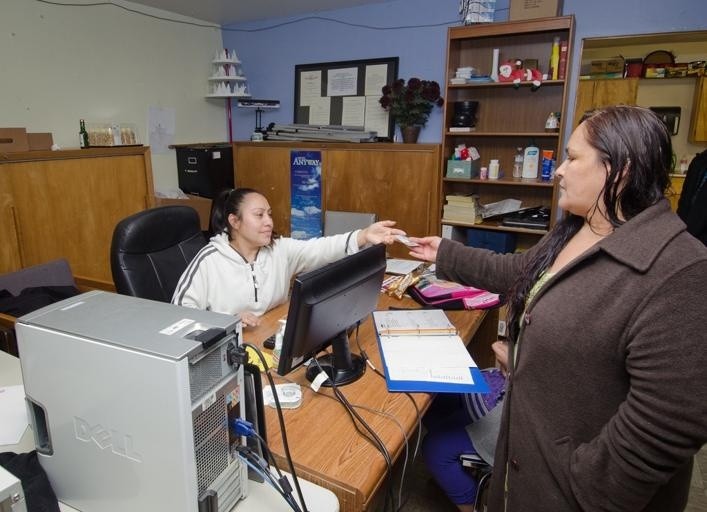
[{"x1": 373, "y1": 308, "x2": 492, "y2": 394}]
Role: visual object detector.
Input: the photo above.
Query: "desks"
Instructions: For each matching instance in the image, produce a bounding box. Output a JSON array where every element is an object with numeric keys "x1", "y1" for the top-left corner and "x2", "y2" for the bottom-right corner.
[
  {"x1": 0, "y1": 148, "x2": 153, "y2": 293},
  {"x1": 232, "y1": 140, "x2": 441, "y2": 241},
  {"x1": 212, "y1": 240, "x2": 500, "y2": 510}
]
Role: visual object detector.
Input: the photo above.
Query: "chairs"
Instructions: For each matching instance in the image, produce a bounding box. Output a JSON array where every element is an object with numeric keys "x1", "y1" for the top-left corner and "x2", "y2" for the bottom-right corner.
[{"x1": 112, "y1": 204, "x2": 209, "y2": 305}]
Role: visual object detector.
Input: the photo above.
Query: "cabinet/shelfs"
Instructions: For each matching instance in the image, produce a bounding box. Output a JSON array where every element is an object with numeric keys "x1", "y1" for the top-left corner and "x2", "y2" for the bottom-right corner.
[
  {"x1": 202, "y1": 57, "x2": 252, "y2": 98},
  {"x1": 173, "y1": 145, "x2": 232, "y2": 235},
  {"x1": 439, "y1": 18, "x2": 575, "y2": 252},
  {"x1": 575, "y1": 31, "x2": 707, "y2": 216}
]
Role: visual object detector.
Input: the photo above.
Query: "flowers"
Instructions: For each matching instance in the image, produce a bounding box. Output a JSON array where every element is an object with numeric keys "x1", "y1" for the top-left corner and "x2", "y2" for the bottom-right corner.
[{"x1": 378, "y1": 74, "x2": 439, "y2": 124}]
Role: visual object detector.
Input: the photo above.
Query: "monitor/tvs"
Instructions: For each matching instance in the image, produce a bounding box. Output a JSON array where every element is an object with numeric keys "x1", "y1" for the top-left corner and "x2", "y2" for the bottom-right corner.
[{"x1": 277, "y1": 243, "x2": 387, "y2": 386}]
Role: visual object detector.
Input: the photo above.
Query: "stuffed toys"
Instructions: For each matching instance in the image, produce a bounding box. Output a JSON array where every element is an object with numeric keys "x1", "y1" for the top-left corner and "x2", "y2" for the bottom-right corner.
[{"x1": 497, "y1": 61, "x2": 544, "y2": 91}]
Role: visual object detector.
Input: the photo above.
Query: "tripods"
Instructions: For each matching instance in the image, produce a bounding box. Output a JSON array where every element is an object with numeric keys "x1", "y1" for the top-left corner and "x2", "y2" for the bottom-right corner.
[{"x1": 261, "y1": 121, "x2": 378, "y2": 143}]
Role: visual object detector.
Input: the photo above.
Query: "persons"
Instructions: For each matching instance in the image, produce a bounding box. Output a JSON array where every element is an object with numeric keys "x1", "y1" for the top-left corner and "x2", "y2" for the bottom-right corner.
[
  {"x1": 418, "y1": 339, "x2": 514, "y2": 511},
  {"x1": 408, "y1": 104, "x2": 706, "y2": 511},
  {"x1": 171, "y1": 189, "x2": 409, "y2": 328}
]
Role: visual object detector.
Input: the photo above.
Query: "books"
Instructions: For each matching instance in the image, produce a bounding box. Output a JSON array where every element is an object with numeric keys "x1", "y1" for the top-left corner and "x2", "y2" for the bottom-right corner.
[{"x1": 439, "y1": 192, "x2": 483, "y2": 225}]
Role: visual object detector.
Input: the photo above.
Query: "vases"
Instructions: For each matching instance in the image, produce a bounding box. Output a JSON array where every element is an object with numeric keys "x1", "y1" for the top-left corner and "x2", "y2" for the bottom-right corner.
[{"x1": 400, "y1": 123, "x2": 421, "y2": 142}]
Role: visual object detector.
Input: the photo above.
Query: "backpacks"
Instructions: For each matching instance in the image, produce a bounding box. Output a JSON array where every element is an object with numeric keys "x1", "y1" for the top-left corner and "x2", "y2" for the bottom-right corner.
[{"x1": 407, "y1": 273, "x2": 506, "y2": 311}]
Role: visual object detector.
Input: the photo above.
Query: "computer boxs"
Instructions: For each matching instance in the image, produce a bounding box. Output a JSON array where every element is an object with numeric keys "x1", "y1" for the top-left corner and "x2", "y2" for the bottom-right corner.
[{"x1": 13, "y1": 289, "x2": 245, "y2": 512}]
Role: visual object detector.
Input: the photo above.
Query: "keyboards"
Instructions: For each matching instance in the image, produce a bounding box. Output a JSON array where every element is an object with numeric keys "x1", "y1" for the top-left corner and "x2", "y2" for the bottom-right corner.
[{"x1": 263, "y1": 334, "x2": 277, "y2": 349}]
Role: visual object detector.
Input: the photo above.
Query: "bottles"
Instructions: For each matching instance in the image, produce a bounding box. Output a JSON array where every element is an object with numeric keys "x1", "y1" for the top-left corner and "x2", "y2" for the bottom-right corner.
[
  {"x1": 679, "y1": 153, "x2": 688, "y2": 174},
  {"x1": 523, "y1": 143, "x2": 540, "y2": 181},
  {"x1": 511, "y1": 146, "x2": 523, "y2": 178},
  {"x1": 479, "y1": 167, "x2": 486, "y2": 180},
  {"x1": 78, "y1": 117, "x2": 90, "y2": 150},
  {"x1": 488, "y1": 159, "x2": 499, "y2": 180}
]
